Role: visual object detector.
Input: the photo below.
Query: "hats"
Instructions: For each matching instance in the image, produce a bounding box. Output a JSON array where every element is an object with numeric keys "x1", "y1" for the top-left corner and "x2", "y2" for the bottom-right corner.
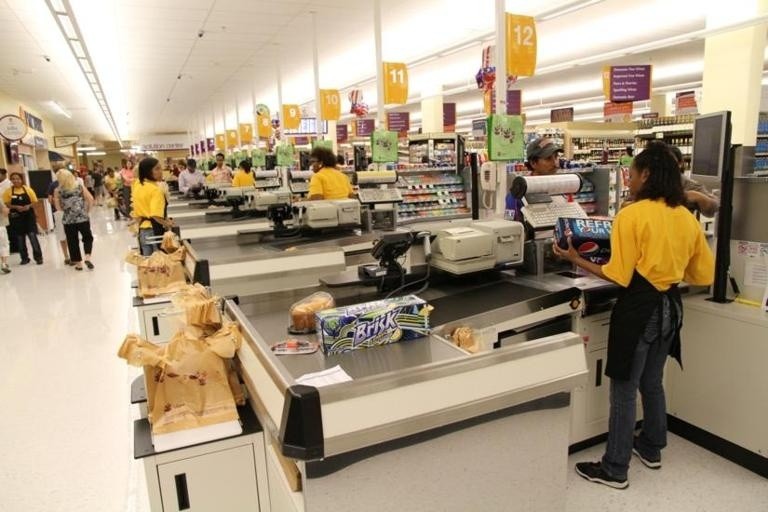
[{"x1": 526, "y1": 137, "x2": 564, "y2": 160}]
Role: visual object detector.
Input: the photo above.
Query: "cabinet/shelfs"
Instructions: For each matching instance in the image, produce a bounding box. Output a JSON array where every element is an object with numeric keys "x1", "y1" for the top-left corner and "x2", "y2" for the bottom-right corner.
[{"x1": 363, "y1": 116, "x2": 767, "y2": 223}]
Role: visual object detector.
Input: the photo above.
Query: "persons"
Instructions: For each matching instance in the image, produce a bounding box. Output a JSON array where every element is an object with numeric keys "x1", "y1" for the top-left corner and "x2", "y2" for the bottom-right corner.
[
  {"x1": 617, "y1": 146, "x2": 635, "y2": 166},
  {"x1": 553, "y1": 144, "x2": 716, "y2": 487},
  {"x1": 508, "y1": 139, "x2": 565, "y2": 214},
  {"x1": 0, "y1": 158, "x2": 140, "y2": 275},
  {"x1": 290, "y1": 147, "x2": 357, "y2": 204},
  {"x1": 166, "y1": 153, "x2": 257, "y2": 197},
  {"x1": 133, "y1": 158, "x2": 176, "y2": 255},
  {"x1": 621, "y1": 145, "x2": 719, "y2": 220}
]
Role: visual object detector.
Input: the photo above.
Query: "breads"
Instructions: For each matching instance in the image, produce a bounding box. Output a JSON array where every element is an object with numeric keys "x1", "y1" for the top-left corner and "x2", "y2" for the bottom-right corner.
[{"x1": 290, "y1": 291, "x2": 336, "y2": 331}]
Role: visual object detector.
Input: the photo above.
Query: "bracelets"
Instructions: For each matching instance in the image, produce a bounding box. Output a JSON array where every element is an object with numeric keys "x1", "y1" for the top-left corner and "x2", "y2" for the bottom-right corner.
[{"x1": 298, "y1": 197, "x2": 302, "y2": 201}]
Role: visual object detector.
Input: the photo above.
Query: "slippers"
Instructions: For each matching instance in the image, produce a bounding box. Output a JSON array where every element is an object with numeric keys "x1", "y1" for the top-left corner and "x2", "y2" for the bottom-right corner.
[
  {"x1": 75, "y1": 265, "x2": 83, "y2": 271},
  {"x1": 85, "y1": 260, "x2": 94, "y2": 269}
]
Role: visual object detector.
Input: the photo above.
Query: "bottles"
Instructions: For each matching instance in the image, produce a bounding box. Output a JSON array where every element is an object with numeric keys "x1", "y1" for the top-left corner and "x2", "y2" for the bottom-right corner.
[{"x1": 571, "y1": 114, "x2": 695, "y2": 169}]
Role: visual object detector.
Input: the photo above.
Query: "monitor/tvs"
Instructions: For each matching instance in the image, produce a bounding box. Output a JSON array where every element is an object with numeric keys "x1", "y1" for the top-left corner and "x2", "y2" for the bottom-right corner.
[
  {"x1": 354, "y1": 144, "x2": 366, "y2": 171},
  {"x1": 265, "y1": 155, "x2": 276, "y2": 170},
  {"x1": 300, "y1": 151, "x2": 310, "y2": 170},
  {"x1": 690, "y1": 110, "x2": 732, "y2": 183}
]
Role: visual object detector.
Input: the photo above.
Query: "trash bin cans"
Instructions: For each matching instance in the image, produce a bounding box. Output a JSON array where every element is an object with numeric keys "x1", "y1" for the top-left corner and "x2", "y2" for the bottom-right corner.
[{"x1": 34, "y1": 198, "x2": 55, "y2": 236}]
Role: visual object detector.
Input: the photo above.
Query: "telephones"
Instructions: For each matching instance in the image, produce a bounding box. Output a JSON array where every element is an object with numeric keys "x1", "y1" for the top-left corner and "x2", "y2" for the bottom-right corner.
[{"x1": 480, "y1": 161, "x2": 497, "y2": 191}]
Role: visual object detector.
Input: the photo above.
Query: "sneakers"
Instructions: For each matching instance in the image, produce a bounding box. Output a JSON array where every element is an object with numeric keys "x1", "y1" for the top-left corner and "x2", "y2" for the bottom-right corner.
[
  {"x1": 631, "y1": 434, "x2": 663, "y2": 468},
  {"x1": 63, "y1": 257, "x2": 76, "y2": 266},
  {"x1": 1, "y1": 263, "x2": 12, "y2": 273},
  {"x1": 36, "y1": 257, "x2": 43, "y2": 265},
  {"x1": 574, "y1": 460, "x2": 629, "y2": 490},
  {"x1": 19, "y1": 257, "x2": 30, "y2": 264}
]
